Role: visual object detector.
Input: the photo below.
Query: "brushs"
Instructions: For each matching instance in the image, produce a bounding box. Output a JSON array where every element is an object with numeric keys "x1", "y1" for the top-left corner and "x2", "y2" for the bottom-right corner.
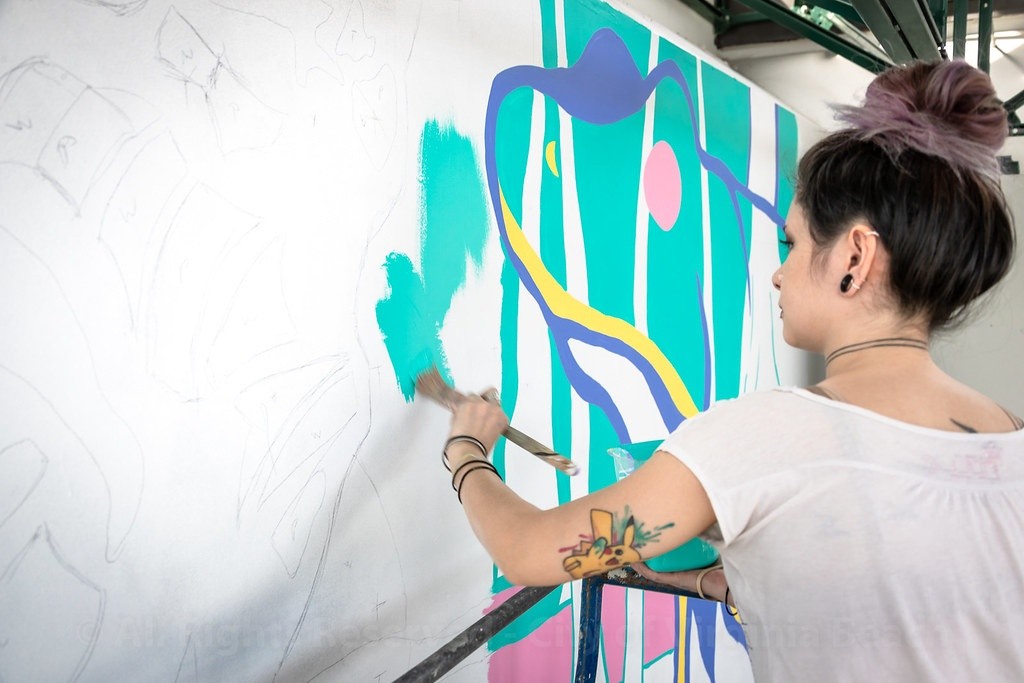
[{"x1": 411, "y1": 363, "x2": 579, "y2": 477}]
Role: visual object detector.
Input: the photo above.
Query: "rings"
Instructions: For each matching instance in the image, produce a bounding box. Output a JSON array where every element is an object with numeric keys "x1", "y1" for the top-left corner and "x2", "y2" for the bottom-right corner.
[{"x1": 481, "y1": 391, "x2": 500, "y2": 402}]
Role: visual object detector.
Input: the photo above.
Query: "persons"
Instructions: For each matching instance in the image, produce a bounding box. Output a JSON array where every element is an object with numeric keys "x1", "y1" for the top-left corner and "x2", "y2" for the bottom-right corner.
[{"x1": 443, "y1": 60, "x2": 1024, "y2": 683}]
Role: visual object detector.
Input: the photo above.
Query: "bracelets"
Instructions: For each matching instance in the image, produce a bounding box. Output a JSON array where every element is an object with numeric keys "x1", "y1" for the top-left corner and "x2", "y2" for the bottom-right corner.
[
  {"x1": 441, "y1": 434, "x2": 504, "y2": 505},
  {"x1": 696, "y1": 565, "x2": 738, "y2": 616}
]
